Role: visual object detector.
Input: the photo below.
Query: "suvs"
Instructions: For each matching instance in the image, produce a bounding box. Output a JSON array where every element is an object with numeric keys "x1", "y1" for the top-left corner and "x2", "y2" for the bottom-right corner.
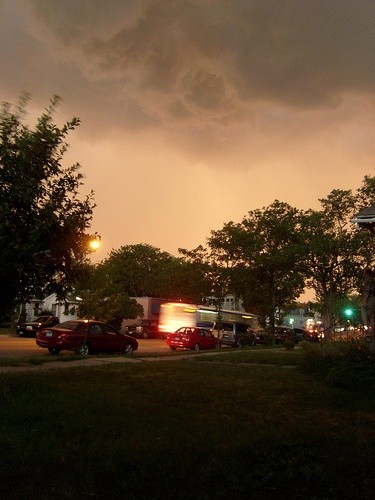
[{"x1": 196, "y1": 321, "x2": 217, "y2": 331}]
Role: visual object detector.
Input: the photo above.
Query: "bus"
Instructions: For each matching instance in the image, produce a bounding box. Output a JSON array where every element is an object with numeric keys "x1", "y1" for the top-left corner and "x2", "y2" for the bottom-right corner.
[{"x1": 158, "y1": 302, "x2": 261, "y2": 333}]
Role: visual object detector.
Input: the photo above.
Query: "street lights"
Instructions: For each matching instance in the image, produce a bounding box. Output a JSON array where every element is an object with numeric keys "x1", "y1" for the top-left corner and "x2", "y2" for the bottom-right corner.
[
  {"x1": 289, "y1": 318, "x2": 294, "y2": 329},
  {"x1": 344, "y1": 308, "x2": 353, "y2": 343}
]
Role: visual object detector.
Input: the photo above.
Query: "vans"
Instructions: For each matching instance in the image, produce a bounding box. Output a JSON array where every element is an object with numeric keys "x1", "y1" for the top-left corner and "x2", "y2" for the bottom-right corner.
[{"x1": 211, "y1": 320, "x2": 255, "y2": 348}]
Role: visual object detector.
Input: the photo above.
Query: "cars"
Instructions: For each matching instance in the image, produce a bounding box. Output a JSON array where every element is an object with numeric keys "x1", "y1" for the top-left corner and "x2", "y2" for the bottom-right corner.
[
  {"x1": 344, "y1": 324, "x2": 368, "y2": 331},
  {"x1": 250, "y1": 326, "x2": 287, "y2": 344},
  {"x1": 165, "y1": 326, "x2": 217, "y2": 350},
  {"x1": 127, "y1": 318, "x2": 171, "y2": 340},
  {"x1": 287, "y1": 328, "x2": 310, "y2": 342},
  {"x1": 16, "y1": 315, "x2": 60, "y2": 337},
  {"x1": 36, "y1": 320, "x2": 139, "y2": 357},
  {"x1": 333, "y1": 326, "x2": 345, "y2": 332}
]
